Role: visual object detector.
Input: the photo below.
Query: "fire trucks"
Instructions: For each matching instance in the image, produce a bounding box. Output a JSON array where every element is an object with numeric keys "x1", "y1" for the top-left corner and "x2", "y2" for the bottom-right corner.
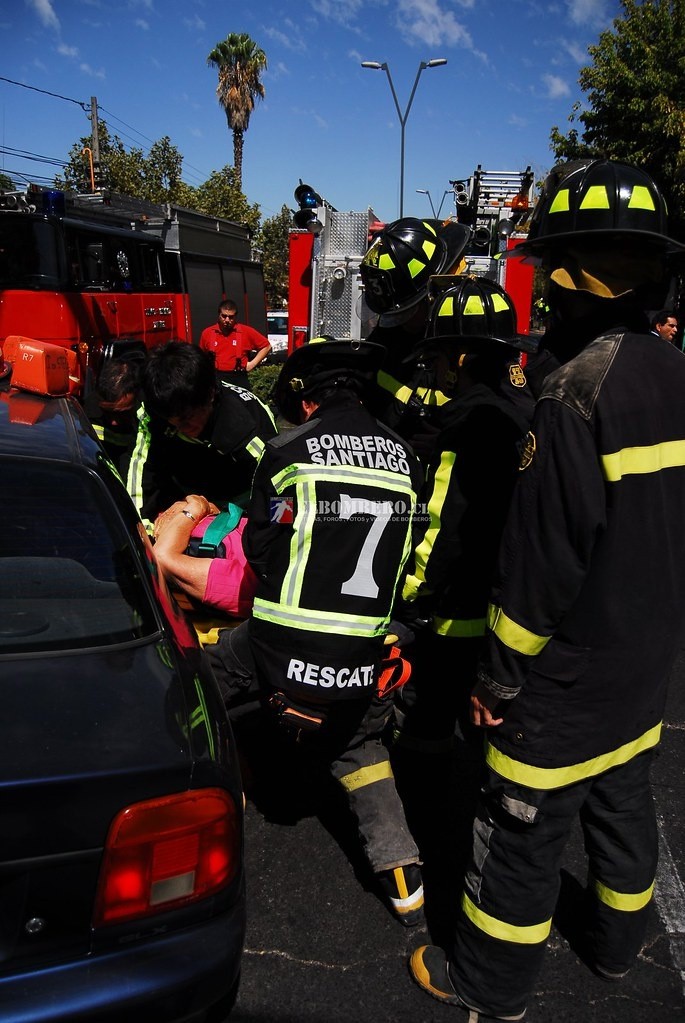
[
  {"x1": 287, "y1": 164, "x2": 557, "y2": 374},
  {"x1": 0, "y1": 176, "x2": 272, "y2": 433}
]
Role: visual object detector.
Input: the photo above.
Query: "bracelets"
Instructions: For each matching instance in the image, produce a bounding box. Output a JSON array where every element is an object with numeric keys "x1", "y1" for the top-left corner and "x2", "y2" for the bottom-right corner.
[{"x1": 182, "y1": 509, "x2": 197, "y2": 523}]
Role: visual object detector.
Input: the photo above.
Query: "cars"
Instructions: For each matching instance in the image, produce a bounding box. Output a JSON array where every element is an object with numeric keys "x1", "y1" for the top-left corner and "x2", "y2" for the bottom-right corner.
[
  {"x1": 263, "y1": 311, "x2": 288, "y2": 363},
  {"x1": 0, "y1": 413, "x2": 247, "y2": 1023}
]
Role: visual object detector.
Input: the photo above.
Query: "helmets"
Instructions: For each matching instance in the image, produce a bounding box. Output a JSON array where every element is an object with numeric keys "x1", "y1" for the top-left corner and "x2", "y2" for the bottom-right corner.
[
  {"x1": 273, "y1": 337, "x2": 387, "y2": 425},
  {"x1": 516, "y1": 159, "x2": 685, "y2": 257},
  {"x1": 359, "y1": 217, "x2": 469, "y2": 316},
  {"x1": 416, "y1": 276, "x2": 544, "y2": 359}
]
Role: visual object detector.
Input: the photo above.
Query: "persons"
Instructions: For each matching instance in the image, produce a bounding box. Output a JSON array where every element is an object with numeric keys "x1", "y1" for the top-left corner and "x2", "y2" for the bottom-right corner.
[{"x1": 83, "y1": 157, "x2": 685, "y2": 1022}]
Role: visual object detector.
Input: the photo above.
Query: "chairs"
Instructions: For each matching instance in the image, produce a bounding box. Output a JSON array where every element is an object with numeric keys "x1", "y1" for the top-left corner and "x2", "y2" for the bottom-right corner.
[{"x1": 1, "y1": 557, "x2": 121, "y2": 600}]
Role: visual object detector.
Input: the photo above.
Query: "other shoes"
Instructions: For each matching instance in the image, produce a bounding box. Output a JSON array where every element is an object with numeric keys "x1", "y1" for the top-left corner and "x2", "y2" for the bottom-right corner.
[
  {"x1": 594, "y1": 959, "x2": 631, "y2": 981},
  {"x1": 409, "y1": 945, "x2": 527, "y2": 1021},
  {"x1": 375, "y1": 863, "x2": 424, "y2": 926}
]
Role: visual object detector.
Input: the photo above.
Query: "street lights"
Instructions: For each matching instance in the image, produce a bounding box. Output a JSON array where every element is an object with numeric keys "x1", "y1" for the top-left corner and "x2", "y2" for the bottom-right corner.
[
  {"x1": 360, "y1": 58, "x2": 448, "y2": 218},
  {"x1": 415, "y1": 190, "x2": 454, "y2": 220}
]
